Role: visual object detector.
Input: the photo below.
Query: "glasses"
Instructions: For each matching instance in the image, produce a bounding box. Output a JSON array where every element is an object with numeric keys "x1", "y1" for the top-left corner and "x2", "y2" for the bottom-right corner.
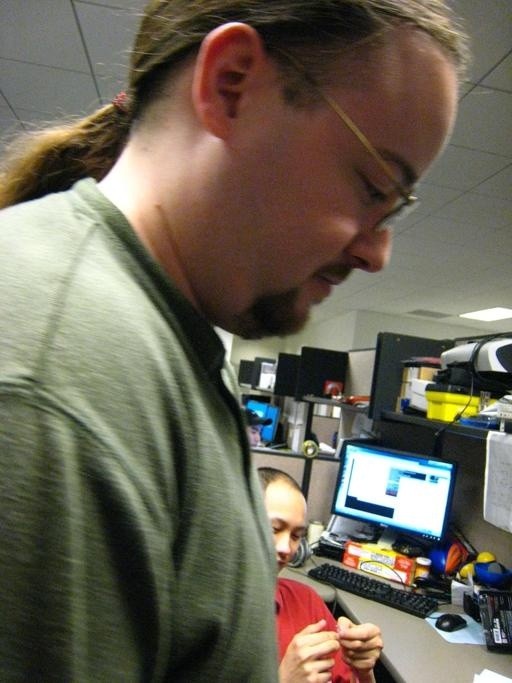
[{"x1": 278, "y1": 46, "x2": 420, "y2": 238}]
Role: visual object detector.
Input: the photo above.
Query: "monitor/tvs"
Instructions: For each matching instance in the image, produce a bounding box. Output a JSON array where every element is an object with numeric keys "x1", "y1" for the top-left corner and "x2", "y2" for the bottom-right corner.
[
  {"x1": 330, "y1": 441, "x2": 459, "y2": 552},
  {"x1": 246, "y1": 399, "x2": 283, "y2": 443}
]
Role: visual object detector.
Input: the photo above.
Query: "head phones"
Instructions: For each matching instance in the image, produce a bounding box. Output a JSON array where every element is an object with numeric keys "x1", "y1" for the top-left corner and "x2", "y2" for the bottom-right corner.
[{"x1": 287, "y1": 537, "x2": 315, "y2": 570}]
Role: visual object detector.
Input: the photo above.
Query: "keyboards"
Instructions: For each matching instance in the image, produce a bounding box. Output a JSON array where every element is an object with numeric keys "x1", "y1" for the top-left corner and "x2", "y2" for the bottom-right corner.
[{"x1": 308, "y1": 562, "x2": 439, "y2": 619}]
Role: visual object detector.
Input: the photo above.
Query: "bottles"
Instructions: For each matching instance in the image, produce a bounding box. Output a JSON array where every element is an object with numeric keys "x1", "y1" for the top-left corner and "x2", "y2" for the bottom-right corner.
[
  {"x1": 410, "y1": 556, "x2": 432, "y2": 595},
  {"x1": 306, "y1": 519, "x2": 325, "y2": 550}
]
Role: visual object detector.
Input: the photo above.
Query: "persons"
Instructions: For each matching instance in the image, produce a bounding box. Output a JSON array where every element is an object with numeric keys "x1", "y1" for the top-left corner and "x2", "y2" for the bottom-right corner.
[
  {"x1": 241, "y1": 408, "x2": 272, "y2": 446},
  {"x1": 0, "y1": 0, "x2": 469, "y2": 683},
  {"x1": 256, "y1": 465, "x2": 384, "y2": 683}
]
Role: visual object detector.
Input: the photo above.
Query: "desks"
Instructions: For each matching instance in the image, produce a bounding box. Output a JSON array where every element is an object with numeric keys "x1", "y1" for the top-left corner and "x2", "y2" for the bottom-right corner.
[{"x1": 277, "y1": 552, "x2": 512, "y2": 683}]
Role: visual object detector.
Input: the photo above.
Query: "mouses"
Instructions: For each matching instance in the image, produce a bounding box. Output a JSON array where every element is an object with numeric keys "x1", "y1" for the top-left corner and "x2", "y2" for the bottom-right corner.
[{"x1": 435, "y1": 613, "x2": 467, "y2": 632}]
